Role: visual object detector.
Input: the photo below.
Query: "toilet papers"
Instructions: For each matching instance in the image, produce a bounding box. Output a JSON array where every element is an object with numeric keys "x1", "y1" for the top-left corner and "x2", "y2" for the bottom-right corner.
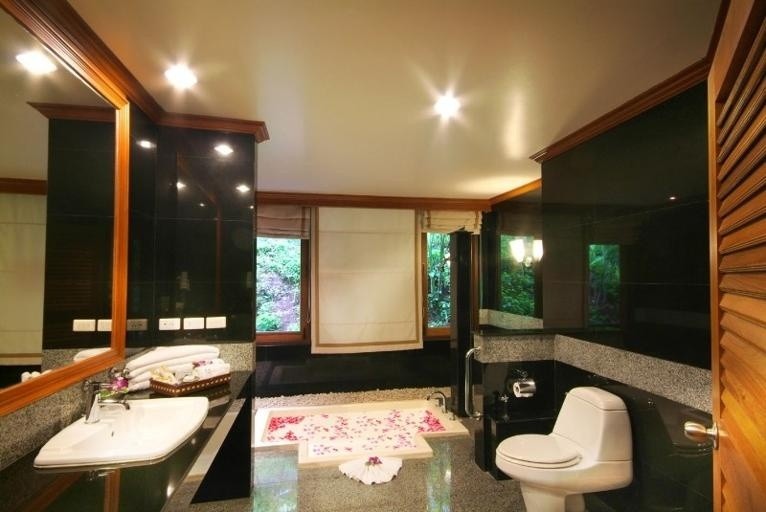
[{"x1": 511, "y1": 380, "x2": 537, "y2": 398}]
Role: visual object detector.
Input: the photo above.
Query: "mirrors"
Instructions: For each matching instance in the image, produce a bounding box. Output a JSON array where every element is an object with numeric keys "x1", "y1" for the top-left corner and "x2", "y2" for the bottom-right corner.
[
  {"x1": 0, "y1": 4, "x2": 128, "y2": 418},
  {"x1": 488, "y1": 178, "x2": 544, "y2": 323}
]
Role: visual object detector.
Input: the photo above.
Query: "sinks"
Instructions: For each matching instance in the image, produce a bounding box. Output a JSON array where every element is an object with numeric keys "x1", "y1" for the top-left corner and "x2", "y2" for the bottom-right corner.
[{"x1": 31, "y1": 396, "x2": 210, "y2": 470}]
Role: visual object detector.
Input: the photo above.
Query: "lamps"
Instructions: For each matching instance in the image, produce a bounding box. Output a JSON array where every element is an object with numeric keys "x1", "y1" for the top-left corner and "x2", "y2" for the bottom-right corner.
[{"x1": 505, "y1": 235, "x2": 544, "y2": 276}]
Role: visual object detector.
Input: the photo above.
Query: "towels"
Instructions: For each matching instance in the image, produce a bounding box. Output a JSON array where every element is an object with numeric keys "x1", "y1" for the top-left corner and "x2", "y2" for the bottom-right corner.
[
  {"x1": 122, "y1": 343, "x2": 231, "y2": 396},
  {"x1": 73, "y1": 347, "x2": 111, "y2": 361}
]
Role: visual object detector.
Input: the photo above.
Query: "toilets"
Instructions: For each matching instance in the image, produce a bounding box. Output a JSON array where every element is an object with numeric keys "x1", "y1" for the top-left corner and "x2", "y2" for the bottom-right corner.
[{"x1": 495, "y1": 386, "x2": 634, "y2": 512}]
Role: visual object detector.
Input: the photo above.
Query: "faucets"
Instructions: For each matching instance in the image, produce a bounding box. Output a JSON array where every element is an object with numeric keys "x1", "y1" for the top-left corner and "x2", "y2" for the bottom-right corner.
[
  {"x1": 426, "y1": 391, "x2": 447, "y2": 415},
  {"x1": 83, "y1": 379, "x2": 131, "y2": 425}
]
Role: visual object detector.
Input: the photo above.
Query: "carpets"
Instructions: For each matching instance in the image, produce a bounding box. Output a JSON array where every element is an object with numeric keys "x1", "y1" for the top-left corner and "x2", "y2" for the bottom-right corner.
[{"x1": 255, "y1": 386, "x2": 451, "y2": 411}]
